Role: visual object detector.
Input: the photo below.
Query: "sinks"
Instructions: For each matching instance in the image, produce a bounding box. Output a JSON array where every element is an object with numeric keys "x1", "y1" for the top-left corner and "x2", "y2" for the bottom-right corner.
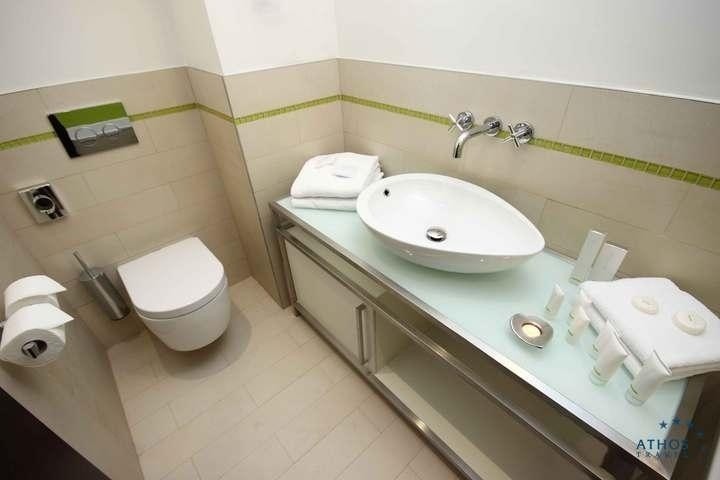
[{"x1": 357, "y1": 172, "x2": 545, "y2": 273}]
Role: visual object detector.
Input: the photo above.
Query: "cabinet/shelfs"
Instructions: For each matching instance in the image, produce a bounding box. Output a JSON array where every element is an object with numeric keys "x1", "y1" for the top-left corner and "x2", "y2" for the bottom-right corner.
[{"x1": 277, "y1": 221, "x2": 646, "y2": 480}]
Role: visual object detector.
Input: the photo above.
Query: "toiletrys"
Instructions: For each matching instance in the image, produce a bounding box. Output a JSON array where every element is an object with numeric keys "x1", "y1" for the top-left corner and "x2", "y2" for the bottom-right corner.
[
  {"x1": 587, "y1": 321, "x2": 620, "y2": 360},
  {"x1": 565, "y1": 305, "x2": 590, "y2": 344},
  {"x1": 542, "y1": 281, "x2": 566, "y2": 321},
  {"x1": 590, "y1": 322, "x2": 626, "y2": 386},
  {"x1": 589, "y1": 337, "x2": 630, "y2": 385},
  {"x1": 676, "y1": 308, "x2": 705, "y2": 335},
  {"x1": 638, "y1": 296, "x2": 656, "y2": 312},
  {"x1": 566, "y1": 291, "x2": 592, "y2": 344},
  {"x1": 570, "y1": 229, "x2": 627, "y2": 281},
  {"x1": 545, "y1": 284, "x2": 564, "y2": 319}
]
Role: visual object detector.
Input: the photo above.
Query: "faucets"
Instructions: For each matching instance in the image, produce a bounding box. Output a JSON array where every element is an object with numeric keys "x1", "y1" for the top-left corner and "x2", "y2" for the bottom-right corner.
[{"x1": 453, "y1": 111, "x2": 503, "y2": 159}]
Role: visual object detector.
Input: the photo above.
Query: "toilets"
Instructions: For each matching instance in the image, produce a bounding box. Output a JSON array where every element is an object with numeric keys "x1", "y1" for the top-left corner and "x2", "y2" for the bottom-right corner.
[{"x1": 117, "y1": 235, "x2": 231, "y2": 352}]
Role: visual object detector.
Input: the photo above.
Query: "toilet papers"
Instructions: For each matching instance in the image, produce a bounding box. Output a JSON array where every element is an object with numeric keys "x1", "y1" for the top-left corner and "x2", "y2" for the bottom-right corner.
[
  {"x1": 0, "y1": 303, "x2": 74, "y2": 368},
  {"x1": 3, "y1": 275, "x2": 66, "y2": 320}
]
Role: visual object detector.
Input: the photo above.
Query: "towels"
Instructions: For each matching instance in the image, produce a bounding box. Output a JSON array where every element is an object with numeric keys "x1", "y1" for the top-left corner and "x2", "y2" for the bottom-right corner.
[
  {"x1": 579, "y1": 278, "x2": 720, "y2": 383},
  {"x1": 290, "y1": 152, "x2": 384, "y2": 211}
]
